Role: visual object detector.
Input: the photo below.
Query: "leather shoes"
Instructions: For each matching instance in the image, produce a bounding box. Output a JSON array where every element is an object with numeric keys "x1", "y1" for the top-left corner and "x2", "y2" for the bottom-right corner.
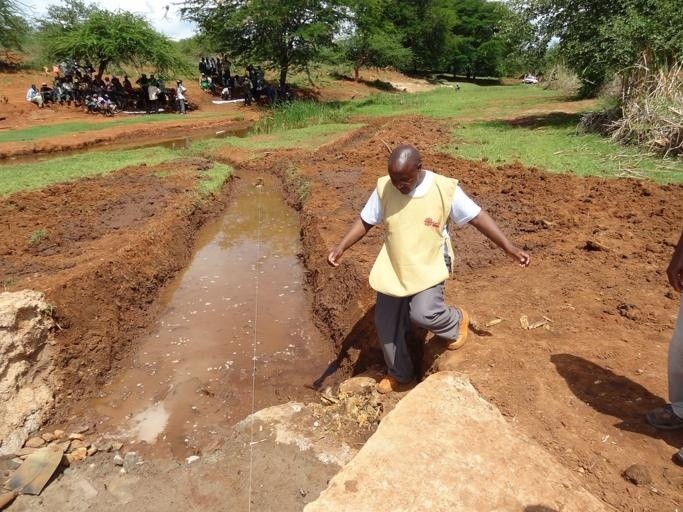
[
  {"x1": 375, "y1": 374, "x2": 401, "y2": 395},
  {"x1": 445, "y1": 305, "x2": 470, "y2": 351}
]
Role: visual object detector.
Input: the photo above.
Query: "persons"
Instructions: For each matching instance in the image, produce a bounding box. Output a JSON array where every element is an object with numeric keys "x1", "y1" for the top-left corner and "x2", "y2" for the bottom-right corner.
[
  {"x1": 328, "y1": 145, "x2": 531, "y2": 395},
  {"x1": 644, "y1": 234, "x2": 682, "y2": 466},
  {"x1": 25, "y1": 55, "x2": 291, "y2": 117}
]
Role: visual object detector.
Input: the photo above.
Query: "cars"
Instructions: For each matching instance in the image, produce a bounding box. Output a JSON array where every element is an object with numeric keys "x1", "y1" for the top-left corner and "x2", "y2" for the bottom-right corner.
[{"x1": 522, "y1": 76, "x2": 538, "y2": 84}]
